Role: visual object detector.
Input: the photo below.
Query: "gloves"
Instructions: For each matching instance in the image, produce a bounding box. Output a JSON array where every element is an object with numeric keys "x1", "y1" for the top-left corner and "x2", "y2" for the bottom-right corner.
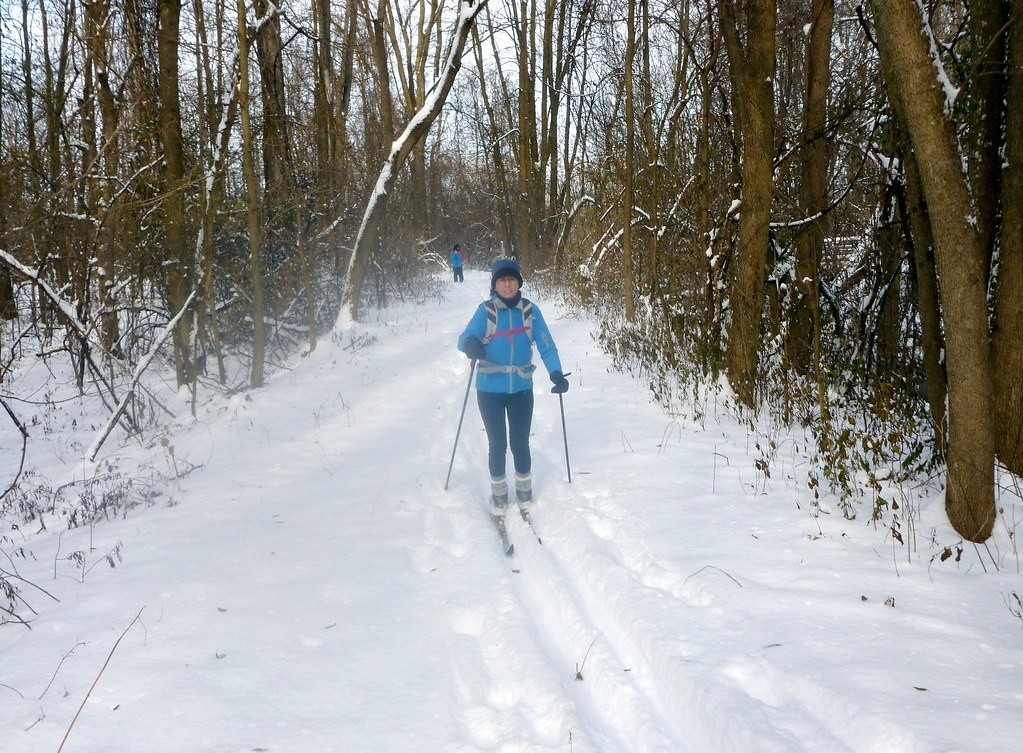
[
  {"x1": 550, "y1": 371, "x2": 569, "y2": 394},
  {"x1": 464, "y1": 336, "x2": 486, "y2": 360}
]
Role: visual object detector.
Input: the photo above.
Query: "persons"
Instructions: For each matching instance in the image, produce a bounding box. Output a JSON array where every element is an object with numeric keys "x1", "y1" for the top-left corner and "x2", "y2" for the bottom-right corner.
[
  {"x1": 450, "y1": 244, "x2": 464, "y2": 283},
  {"x1": 458, "y1": 259, "x2": 569, "y2": 514}
]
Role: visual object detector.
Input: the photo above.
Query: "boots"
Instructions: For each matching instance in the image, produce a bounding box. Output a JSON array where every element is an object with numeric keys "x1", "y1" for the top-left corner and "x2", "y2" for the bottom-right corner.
[
  {"x1": 490, "y1": 475, "x2": 508, "y2": 508},
  {"x1": 515, "y1": 470, "x2": 532, "y2": 502}
]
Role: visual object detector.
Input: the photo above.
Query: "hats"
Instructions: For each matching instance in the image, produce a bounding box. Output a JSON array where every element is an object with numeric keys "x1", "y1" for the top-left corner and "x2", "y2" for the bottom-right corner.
[{"x1": 491, "y1": 259, "x2": 523, "y2": 290}]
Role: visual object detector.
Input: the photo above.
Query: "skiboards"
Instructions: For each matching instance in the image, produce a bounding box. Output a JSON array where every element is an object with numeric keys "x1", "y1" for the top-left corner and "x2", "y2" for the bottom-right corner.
[{"x1": 490, "y1": 494, "x2": 544, "y2": 556}]
